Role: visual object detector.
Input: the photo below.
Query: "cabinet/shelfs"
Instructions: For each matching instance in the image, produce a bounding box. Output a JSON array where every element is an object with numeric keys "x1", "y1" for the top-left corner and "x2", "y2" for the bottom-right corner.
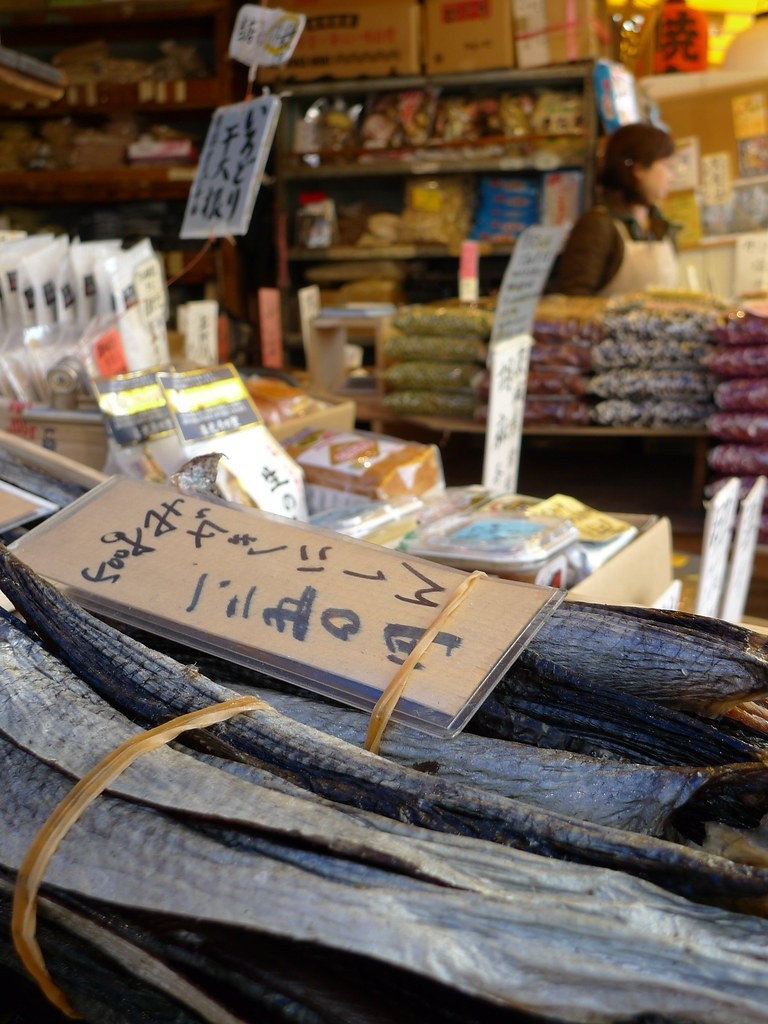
[
  {"x1": 0, "y1": 0, "x2": 237, "y2": 325},
  {"x1": 266, "y1": 58, "x2": 598, "y2": 282}
]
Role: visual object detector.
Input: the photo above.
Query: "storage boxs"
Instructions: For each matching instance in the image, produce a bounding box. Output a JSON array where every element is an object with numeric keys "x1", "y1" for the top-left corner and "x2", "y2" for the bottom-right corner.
[
  {"x1": 255, "y1": 1, "x2": 616, "y2": 89},
  {"x1": 5, "y1": 360, "x2": 673, "y2": 615}
]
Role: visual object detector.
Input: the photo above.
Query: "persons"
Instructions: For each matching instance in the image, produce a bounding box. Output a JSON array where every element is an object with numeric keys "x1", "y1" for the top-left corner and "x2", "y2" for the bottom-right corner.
[{"x1": 544, "y1": 125, "x2": 681, "y2": 295}]
